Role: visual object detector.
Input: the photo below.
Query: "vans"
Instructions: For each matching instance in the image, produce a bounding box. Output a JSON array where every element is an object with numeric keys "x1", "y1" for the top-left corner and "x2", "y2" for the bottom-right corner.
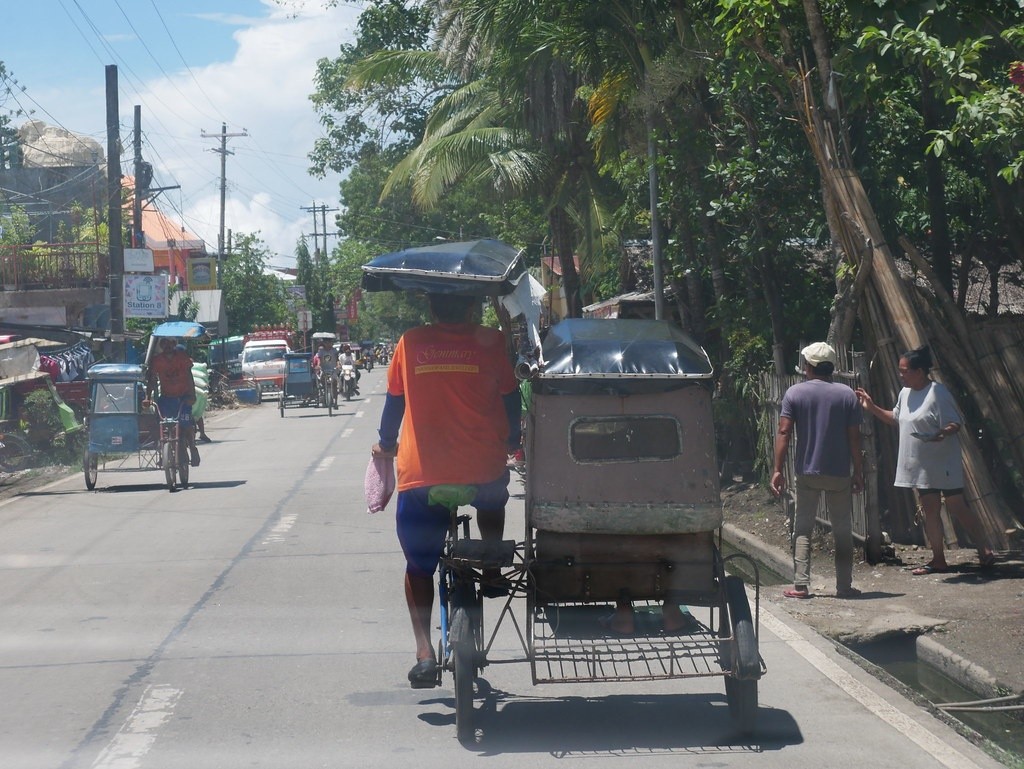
[{"x1": 238, "y1": 339, "x2": 294, "y2": 389}]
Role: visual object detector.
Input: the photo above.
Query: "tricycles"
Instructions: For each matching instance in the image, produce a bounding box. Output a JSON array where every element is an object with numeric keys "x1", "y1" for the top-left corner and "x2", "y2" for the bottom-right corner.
[
  {"x1": 281, "y1": 333, "x2": 343, "y2": 420},
  {"x1": 83, "y1": 363, "x2": 193, "y2": 496},
  {"x1": 369, "y1": 316, "x2": 766, "y2": 746}
]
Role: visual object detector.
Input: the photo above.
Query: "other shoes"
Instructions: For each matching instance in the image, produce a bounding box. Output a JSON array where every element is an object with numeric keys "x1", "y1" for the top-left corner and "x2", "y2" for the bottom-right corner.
[
  {"x1": 156, "y1": 459, "x2": 162, "y2": 467},
  {"x1": 190, "y1": 448, "x2": 200, "y2": 467},
  {"x1": 408, "y1": 658, "x2": 437, "y2": 682},
  {"x1": 333, "y1": 404, "x2": 338, "y2": 410},
  {"x1": 199, "y1": 435, "x2": 211, "y2": 442},
  {"x1": 480, "y1": 576, "x2": 512, "y2": 598}
]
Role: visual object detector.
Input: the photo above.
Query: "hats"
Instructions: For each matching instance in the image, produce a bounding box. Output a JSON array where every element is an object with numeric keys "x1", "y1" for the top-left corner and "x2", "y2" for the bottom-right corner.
[
  {"x1": 800, "y1": 341, "x2": 836, "y2": 366},
  {"x1": 158, "y1": 336, "x2": 177, "y2": 343}
]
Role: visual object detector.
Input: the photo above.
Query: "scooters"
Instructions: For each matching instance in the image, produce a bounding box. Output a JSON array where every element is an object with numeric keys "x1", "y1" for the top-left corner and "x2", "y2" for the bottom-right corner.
[
  {"x1": 338, "y1": 360, "x2": 361, "y2": 402},
  {"x1": 364, "y1": 353, "x2": 373, "y2": 372},
  {"x1": 379, "y1": 354, "x2": 388, "y2": 366}
]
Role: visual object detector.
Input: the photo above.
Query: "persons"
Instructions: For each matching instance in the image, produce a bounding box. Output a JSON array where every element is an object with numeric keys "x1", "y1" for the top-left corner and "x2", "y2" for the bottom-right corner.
[
  {"x1": 362, "y1": 346, "x2": 374, "y2": 369},
  {"x1": 142, "y1": 337, "x2": 211, "y2": 467},
  {"x1": 311, "y1": 339, "x2": 340, "y2": 410},
  {"x1": 105, "y1": 384, "x2": 146, "y2": 411},
  {"x1": 372, "y1": 292, "x2": 521, "y2": 682},
  {"x1": 374, "y1": 346, "x2": 393, "y2": 359},
  {"x1": 855, "y1": 344, "x2": 996, "y2": 576},
  {"x1": 770, "y1": 342, "x2": 864, "y2": 598},
  {"x1": 338, "y1": 345, "x2": 361, "y2": 396}
]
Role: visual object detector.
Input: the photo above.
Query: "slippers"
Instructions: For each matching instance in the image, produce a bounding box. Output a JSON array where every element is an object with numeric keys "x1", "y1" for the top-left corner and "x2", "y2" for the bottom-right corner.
[
  {"x1": 594, "y1": 614, "x2": 638, "y2": 638},
  {"x1": 977, "y1": 553, "x2": 996, "y2": 571},
  {"x1": 837, "y1": 588, "x2": 861, "y2": 598},
  {"x1": 663, "y1": 613, "x2": 697, "y2": 635},
  {"x1": 912, "y1": 563, "x2": 949, "y2": 574},
  {"x1": 783, "y1": 589, "x2": 808, "y2": 599}
]
